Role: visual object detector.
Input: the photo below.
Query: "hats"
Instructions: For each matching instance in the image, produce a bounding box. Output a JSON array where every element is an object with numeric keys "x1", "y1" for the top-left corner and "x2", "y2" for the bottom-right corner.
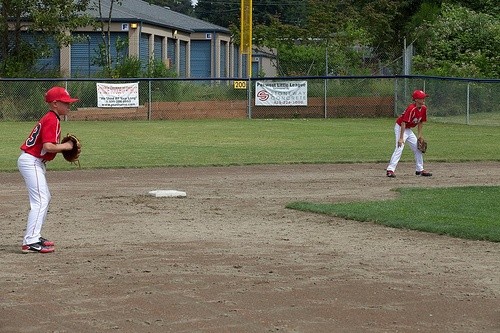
[
  {"x1": 44, "y1": 87, "x2": 80, "y2": 103},
  {"x1": 412, "y1": 90, "x2": 430, "y2": 100}
]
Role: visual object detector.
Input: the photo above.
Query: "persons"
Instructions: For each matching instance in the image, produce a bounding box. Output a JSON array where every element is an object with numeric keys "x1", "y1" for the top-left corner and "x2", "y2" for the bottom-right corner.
[
  {"x1": 18, "y1": 86, "x2": 81, "y2": 254},
  {"x1": 386, "y1": 90, "x2": 433, "y2": 177}
]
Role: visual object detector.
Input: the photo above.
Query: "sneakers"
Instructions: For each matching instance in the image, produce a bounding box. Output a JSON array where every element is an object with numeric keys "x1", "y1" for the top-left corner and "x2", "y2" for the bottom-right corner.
[
  {"x1": 21, "y1": 237, "x2": 55, "y2": 254},
  {"x1": 386, "y1": 171, "x2": 396, "y2": 177},
  {"x1": 416, "y1": 170, "x2": 432, "y2": 177}
]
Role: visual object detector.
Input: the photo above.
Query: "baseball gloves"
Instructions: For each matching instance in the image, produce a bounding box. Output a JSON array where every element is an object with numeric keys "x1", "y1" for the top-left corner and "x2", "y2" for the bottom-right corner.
[
  {"x1": 417, "y1": 137, "x2": 428, "y2": 153},
  {"x1": 61, "y1": 132, "x2": 82, "y2": 168}
]
